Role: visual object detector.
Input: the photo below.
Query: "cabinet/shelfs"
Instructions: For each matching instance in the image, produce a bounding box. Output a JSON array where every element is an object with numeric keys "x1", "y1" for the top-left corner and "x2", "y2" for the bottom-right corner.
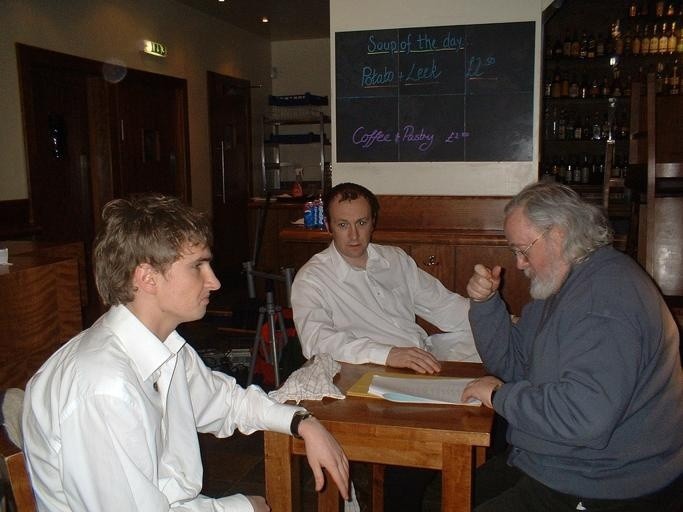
[
  {"x1": 539, "y1": 94, "x2": 628, "y2": 196},
  {"x1": 257, "y1": 92, "x2": 331, "y2": 195},
  {"x1": 272, "y1": 221, "x2": 533, "y2": 332}
]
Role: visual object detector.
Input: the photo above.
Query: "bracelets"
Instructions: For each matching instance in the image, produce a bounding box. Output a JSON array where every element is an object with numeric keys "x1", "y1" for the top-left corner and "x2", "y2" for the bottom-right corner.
[{"x1": 490, "y1": 383, "x2": 504, "y2": 411}]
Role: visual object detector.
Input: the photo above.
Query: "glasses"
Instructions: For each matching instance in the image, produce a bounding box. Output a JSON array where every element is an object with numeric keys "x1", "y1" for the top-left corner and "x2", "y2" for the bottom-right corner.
[{"x1": 510, "y1": 224, "x2": 554, "y2": 255}]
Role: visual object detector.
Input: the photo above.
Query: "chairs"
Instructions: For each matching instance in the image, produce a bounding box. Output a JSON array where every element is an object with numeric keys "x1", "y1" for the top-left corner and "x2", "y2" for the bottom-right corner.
[{"x1": 0, "y1": 438, "x2": 38, "y2": 512}]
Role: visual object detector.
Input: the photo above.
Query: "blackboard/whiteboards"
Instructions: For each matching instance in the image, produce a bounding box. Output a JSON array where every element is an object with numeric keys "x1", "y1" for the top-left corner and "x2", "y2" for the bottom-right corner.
[{"x1": 335, "y1": 21, "x2": 537, "y2": 163}]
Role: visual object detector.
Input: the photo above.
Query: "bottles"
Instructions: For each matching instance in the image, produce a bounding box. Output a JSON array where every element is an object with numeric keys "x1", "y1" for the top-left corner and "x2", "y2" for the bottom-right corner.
[{"x1": 545, "y1": 0, "x2": 683, "y2": 184}]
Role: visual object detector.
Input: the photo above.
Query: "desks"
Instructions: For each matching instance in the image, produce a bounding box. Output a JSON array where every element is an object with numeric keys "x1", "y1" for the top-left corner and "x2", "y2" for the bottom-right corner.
[{"x1": 259, "y1": 316, "x2": 509, "y2": 512}]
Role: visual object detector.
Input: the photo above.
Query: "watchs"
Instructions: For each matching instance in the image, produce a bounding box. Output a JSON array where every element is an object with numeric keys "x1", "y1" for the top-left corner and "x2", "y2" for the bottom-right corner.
[{"x1": 290, "y1": 409, "x2": 313, "y2": 439}]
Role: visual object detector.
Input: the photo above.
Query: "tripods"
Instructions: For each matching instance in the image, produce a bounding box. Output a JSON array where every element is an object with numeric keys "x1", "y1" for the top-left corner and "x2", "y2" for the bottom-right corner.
[{"x1": 242, "y1": 276, "x2": 295, "y2": 390}]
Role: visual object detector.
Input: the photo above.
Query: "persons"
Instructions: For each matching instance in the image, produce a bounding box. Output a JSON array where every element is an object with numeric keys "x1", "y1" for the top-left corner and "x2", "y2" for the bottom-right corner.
[
  {"x1": 287, "y1": 182, "x2": 500, "y2": 376},
  {"x1": 458, "y1": 181, "x2": 682, "y2": 509},
  {"x1": 15, "y1": 194, "x2": 353, "y2": 511}
]
